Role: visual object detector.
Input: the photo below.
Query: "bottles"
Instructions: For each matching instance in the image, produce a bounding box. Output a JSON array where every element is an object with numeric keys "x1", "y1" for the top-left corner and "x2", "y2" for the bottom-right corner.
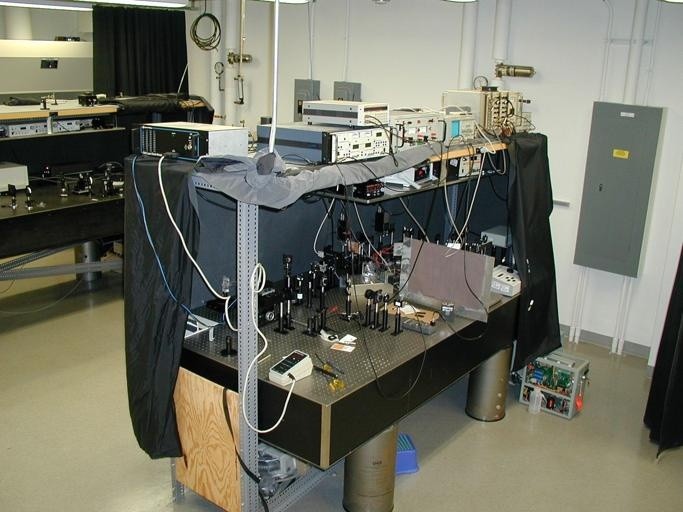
[{"x1": 527, "y1": 386, "x2": 542, "y2": 414}]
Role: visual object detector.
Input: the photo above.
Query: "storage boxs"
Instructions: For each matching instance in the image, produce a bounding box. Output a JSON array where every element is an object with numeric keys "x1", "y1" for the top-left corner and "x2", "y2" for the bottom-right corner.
[{"x1": 518, "y1": 351, "x2": 589, "y2": 421}]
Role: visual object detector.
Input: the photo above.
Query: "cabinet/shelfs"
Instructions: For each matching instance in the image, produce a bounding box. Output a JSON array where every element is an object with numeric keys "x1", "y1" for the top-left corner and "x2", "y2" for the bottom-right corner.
[
  {"x1": 127, "y1": 129, "x2": 551, "y2": 512},
  {"x1": 0, "y1": 90, "x2": 216, "y2": 298}
]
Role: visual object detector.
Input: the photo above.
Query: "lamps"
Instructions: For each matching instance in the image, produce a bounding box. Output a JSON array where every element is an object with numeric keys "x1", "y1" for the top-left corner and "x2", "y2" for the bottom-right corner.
[
  {"x1": 97, "y1": 1, "x2": 193, "y2": 10},
  {"x1": 0, "y1": 0, "x2": 92, "y2": 14}
]
